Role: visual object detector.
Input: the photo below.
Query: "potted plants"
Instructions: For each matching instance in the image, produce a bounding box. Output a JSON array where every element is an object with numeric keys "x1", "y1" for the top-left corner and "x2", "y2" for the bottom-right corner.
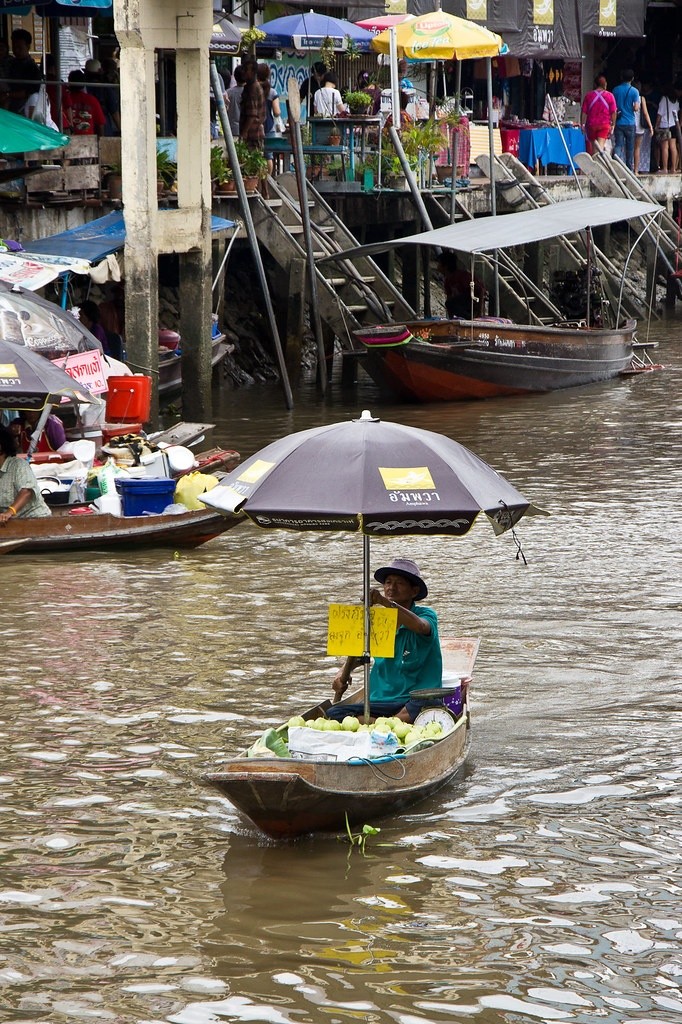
[
  {"x1": 398, "y1": 128, "x2": 442, "y2": 189},
  {"x1": 236, "y1": 143, "x2": 269, "y2": 194},
  {"x1": 214, "y1": 158, "x2": 237, "y2": 195},
  {"x1": 306, "y1": 154, "x2": 329, "y2": 178},
  {"x1": 157, "y1": 151, "x2": 175, "y2": 197},
  {"x1": 389, "y1": 168, "x2": 405, "y2": 189},
  {"x1": 328, "y1": 127, "x2": 340, "y2": 146},
  {"x1": 344, "y1": 90, "x2": 372, "y2": 113},
  {"x1": 110, "y1": 164, "x2": 121, "y2": 197}
]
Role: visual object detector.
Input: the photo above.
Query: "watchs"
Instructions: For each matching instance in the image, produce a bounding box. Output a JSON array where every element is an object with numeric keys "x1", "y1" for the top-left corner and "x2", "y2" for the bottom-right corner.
[{"x1": 391, "y1": 602, "x2": 397, "y2": 608}]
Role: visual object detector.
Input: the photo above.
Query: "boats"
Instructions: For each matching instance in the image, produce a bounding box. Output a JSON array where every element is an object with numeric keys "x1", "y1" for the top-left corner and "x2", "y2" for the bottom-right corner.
[
  {"x1": 201, "y1": 675, "x2": 474, "y2": 841},
  {"x1": 314, "y1": 197, "x2": 666, "y2": 402},
  {"x1": 0, "y1": 421, "x2": 249, "y2": 556}
]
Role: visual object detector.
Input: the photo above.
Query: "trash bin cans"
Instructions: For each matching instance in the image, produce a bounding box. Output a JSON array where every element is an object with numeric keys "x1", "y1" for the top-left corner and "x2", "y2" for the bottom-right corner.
[{"x1": 115, "y1": 476, "x2": 177, "y2": 517}]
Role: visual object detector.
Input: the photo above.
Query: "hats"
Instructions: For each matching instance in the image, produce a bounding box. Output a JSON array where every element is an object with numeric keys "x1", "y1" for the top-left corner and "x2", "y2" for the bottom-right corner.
[{"x1": 374, "y1": 559, "x2": 428, "y2": 602}]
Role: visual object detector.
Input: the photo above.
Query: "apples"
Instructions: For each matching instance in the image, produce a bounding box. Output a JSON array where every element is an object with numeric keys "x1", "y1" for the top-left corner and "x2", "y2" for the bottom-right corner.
[{"x1": 286, "y1": 715, "x2": 442, "y2": 746}]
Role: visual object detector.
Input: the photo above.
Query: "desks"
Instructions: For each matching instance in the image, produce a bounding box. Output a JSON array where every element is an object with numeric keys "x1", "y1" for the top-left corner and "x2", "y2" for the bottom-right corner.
[
  {"x1": 608, "y1": 129, "x2": 652, "y2": 172},
  {"x1": 307, "y1": 117, "x2": 382, "y2": 186},
  {"x1": 470, "y1": 128, "x2": 503, "y2": 166},
  {"x1": 268, "y1": 145, "x2": 346, "y2": 179},
  {"x1": 521, "y1": 126, "x2": 587, "y2": 175},
  {"x1": 501, "y1": 129, "x2": 592, "y2": 175}
]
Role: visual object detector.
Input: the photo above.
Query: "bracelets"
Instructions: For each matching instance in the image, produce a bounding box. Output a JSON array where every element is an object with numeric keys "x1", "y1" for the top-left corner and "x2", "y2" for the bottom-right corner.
[{"x1": 8, "y1": 506, "x2": 16, "y2": 516}]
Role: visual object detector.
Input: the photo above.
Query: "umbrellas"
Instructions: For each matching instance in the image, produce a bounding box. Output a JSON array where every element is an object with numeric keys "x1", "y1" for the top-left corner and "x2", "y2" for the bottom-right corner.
[
  {"x1": 0, "y1": 341, "x2": 103, "y2": 411},
  {"x1": 252, "y1": 10, "x2": 378, "y2": 133},
  {"x1": 0, "y1": 278, "x2": 103, "y2": 351},
  {"x1": 355, "y1": 13, "x2": 418, "y2": 35},
  {"x1": 372, "y1": 8, "x2": 504, "y2": 147},
  {"x1": 0, "y1": 108, "x2": 71, "y2": 152},
  {"x1": 196, "y1": 410, "x2": 551, "y2": 722}
]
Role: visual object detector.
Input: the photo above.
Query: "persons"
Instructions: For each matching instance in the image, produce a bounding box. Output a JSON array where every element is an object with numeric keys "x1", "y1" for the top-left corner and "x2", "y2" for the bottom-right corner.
[
  {"x1": 0, "y1": 425, "x2": 54, "y2": 523},
  {"x1": 0, "y1": 29, "x2": 121, "y2": 137},
  {"x1": 211, "y1": 59, "x2": 414, "y2": 178},
  {"x1": 582, "y1": 71, "x2": 682, "y2": 174},
  {"x1": 8, "y1": 409, "x2": 94, "y2": 503},
  {"x1": 325, "y1": 559, "x2": 442, "y2": 722}
]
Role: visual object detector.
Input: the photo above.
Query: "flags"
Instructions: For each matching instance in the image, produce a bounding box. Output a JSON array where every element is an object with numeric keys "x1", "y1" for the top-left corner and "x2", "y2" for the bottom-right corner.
[{"x1": 543, "y1": 96, "x2": 555, "y2": 121}]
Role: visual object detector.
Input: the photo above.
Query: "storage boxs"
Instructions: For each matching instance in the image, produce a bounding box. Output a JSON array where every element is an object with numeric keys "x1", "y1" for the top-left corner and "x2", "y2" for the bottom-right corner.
[{"x1": 115, "y1": 476, "x2": 176, "y2": 516}]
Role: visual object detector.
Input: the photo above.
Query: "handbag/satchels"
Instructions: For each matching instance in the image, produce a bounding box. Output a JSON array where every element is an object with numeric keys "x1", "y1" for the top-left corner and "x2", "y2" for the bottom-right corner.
[
  {"x1": 286, "y1": 727, "x2": 371, "y2": 763},
  {"x1": 640, "y1": 96, "x2": 649, "y2": 129},
  {"x1": 655, "y1": 127, "x2": 672, "y2": 141},
  {"x1": 616, "y1": 109, "x2": 621, "y2": 118}
]
